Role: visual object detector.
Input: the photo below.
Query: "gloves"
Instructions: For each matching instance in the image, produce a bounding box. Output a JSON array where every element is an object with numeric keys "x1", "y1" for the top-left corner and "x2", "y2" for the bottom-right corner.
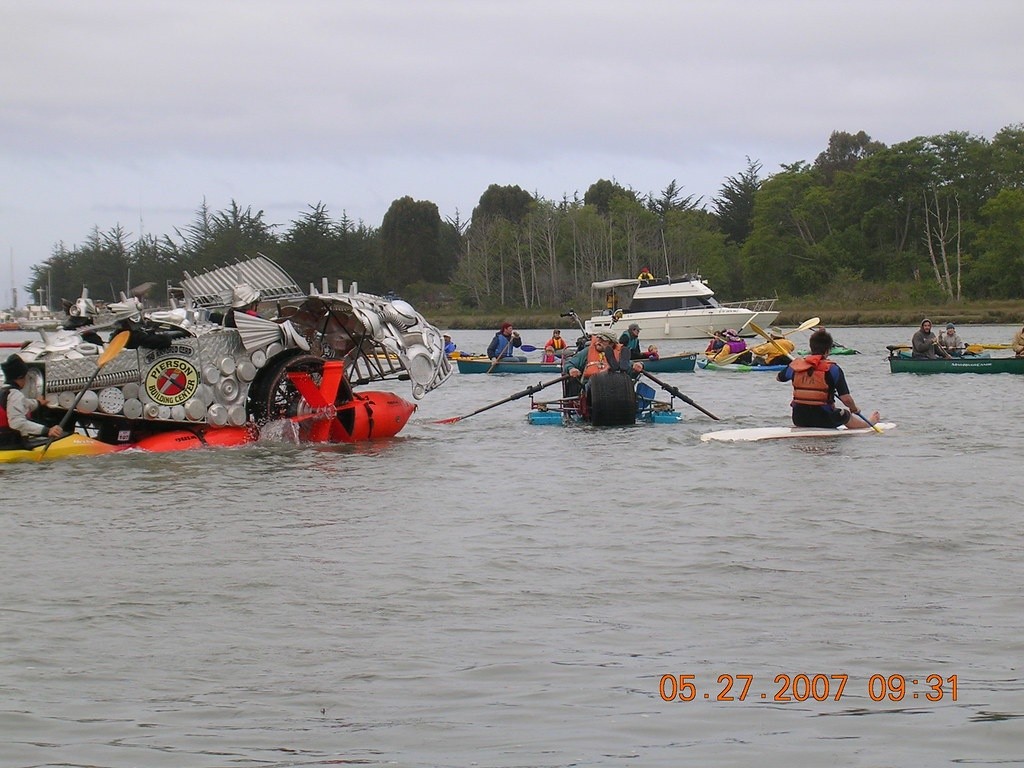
[
  {"x1": 513, "y1": 330, "x2": 520, "y2": 338},
  {"x1": 491, "y1": 358, "x2": 498, "y2": 365}
]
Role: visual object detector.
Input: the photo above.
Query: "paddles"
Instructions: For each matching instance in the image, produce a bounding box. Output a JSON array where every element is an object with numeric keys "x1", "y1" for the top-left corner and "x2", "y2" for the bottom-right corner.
[
  {"x1": 718, "y1": 317, "x2": 821, "y2": 365},
  {"x1": 36, "y1": 329, "x2": 132, "y2": 463},
  {"x1": 799, "y1": 321, "x2": 861, "y2": 355},
  {"x1": 897, "y1": 344, "x2": 983, "y2": 353},
  {"x1": 449, "y1": 351, "x2": 461, "y2": 358},
  {"x1": 521, "y1": 345, "x2": 546, "y2": 353},
  {"x1": 749, "y1": 321, "x2": 884, "y2": 434},
  {"x1": 703, "y1": 312, "x2": 759, "y2": 369},
  {"x1": 630, "y1": 360, "x2": 721, "y2": 420},
  {"x1": 935, "y1": 341, "x2": 952, "y2": 359},
  {"x1": 694, "y1": 326, "x2": 728, "y2": 343},
  {"x1": 435, "y1": 370, "x2": 582, "y2": 424},
  {"x1": 487, "y1": 332, "x2": 519, "y2": 373}
]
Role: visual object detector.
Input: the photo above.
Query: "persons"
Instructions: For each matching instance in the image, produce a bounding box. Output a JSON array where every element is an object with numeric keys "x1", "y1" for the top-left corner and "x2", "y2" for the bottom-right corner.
[
  {"x1": 705, "y1": 328, "x2": 748, "y2": 364},
  {"x1": 545, "y1": 329, "x2": 567, "y2": 351},
  {"x1": 911, "y1": 318, "x2": 951, "y2": 361},
  {"x1": 751, "y1": 326, "x2": 794, "y2": 366},
  {"x1": 1012, "y1": 326, "x2": 1024, "y2": 359},
  {"x1": 619, "y1": 323, "x2": 647, "y2": 359},
  {"x1": 209, "y1": 282, "x2": 272, "y2": 328},
  {"x1": 638, "y1": 267, "x2": 654, "y2": 282},
  {"x1": 562, "y1": 331, "x2": 645, "y2": 399},
  {"x1": 939, "y1": 322, "x2": 967, "y2": 355},
  {"x1": 487, "y1": 322, "x2": 528, "y2": 365},
  {"x1": 443, "y1": 334, "x2": 456, "y2": 353},
  {"x1": 606, "y1": 291, "x2": 618, "y2": 311},
  {"x1": 776, "y1": 330, "x2": 882, "y2": 428},
  {"x1": 0, "y1": 354, "x2": 63, "y2": 440},
  {"x1": 648, "y1": 345, "x2": 658, "y2": 359},
  {"x1": 541, "y1": 346, "x2": 561, "y2": 364}
]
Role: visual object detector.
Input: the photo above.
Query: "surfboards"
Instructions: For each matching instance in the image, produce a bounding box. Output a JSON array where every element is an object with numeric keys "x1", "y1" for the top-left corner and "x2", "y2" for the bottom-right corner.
[{"x1": 701, "y1": 422, "x2": 898, "y2": 443}]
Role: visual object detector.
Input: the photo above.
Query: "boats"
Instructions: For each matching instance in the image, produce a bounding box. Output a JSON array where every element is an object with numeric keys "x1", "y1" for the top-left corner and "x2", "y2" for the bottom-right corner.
[
  {"x1": 889, "y1": 348, "x2": 1024, "y2": 375},
  {"x1": 529, "y1": 310, "x2": 684, "y2": 425},
  {"x1": 584, "y1": 275, "x2": 781, "y2": 340},
  {"x1": 697, "y1": 359, "x2": 789, "y2": 371},
  {"x1": 0, "y1": 251, "x2": 455, "y2": 464},
  {"x1": 798, "y1": 348, "x2": 855, "y2": 355},
  {"x1": 366, "y1": 353, "x2": 488, "y2": 360},
  {"x1": 457, "y1": 352, "x2": 699, "y2": 374}
]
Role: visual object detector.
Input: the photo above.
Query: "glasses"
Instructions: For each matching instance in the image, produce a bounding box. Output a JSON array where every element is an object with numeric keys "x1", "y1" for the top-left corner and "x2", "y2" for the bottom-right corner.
[{"x1": 555, "y1": 332, "x2": 559, "y2": 334}]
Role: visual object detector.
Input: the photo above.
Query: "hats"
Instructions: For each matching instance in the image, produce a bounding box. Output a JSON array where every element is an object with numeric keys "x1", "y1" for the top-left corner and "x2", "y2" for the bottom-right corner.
[
  {"x1": 723, "y1": 329, "x2": 740, "y2": 341},
  {"x1": 443, "y1": 333, "x2": 451, "y2": 338},
  {"x1": 946, "y1": 323, "x2": 955, "y2": 329},
  {"x1": 767, "y1": 327, "x2": 784, "y2": 338},
  {"x1": 546, "y1": 346, "x2": 555, "y2": 351},
  {"x1": 553, "y1": 329, "x2": 561, "y2": 333},
  {"x1": 595, "y1": 331, "x2": 619, "y2": 343},
  {"x1": 629, "y1": 323, "x2": 643, "y2": 331},
  {"x1": 502, "y1": 322, "x2": 513, "y2": 328},
  {"x1": 0, "y1": 353, "x2": 30, "y2": 380}
]
